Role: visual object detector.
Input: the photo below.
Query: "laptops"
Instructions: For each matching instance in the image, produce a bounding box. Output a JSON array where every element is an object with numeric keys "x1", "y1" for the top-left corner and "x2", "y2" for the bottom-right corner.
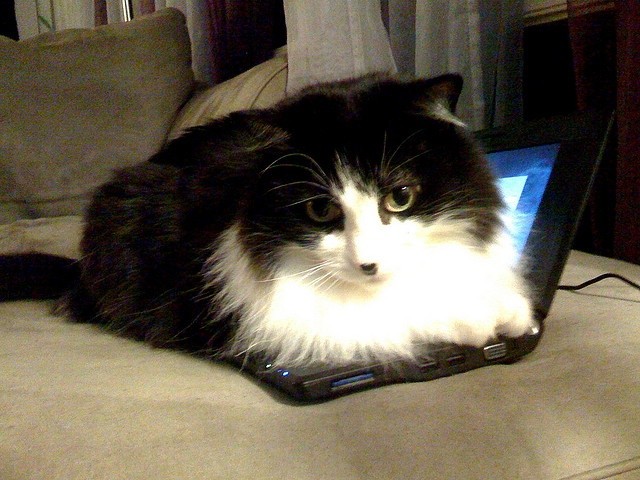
[{"x1": 225, "y1": 108, "x2": 618, "y2": 401}]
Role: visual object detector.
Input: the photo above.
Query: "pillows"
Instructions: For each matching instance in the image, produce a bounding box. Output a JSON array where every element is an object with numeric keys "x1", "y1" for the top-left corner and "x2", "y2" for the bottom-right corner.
[
  {"x1": 163, "y1": 56, "x2": 287, "y2": 144},
  {"x1": 0, "y1": 6, "x2": 195, "y2": 221}
]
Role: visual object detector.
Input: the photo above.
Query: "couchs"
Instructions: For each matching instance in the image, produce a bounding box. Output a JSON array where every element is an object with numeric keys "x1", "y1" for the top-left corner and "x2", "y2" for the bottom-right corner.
[{"x1": 0, "y1": 217, "x2": 640, "y2": 480}]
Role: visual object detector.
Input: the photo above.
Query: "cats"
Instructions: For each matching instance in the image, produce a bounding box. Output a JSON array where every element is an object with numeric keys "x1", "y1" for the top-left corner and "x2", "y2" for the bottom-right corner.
[{"x1": 0, "y1": 72, "x2": 534, "y2": 380}]
